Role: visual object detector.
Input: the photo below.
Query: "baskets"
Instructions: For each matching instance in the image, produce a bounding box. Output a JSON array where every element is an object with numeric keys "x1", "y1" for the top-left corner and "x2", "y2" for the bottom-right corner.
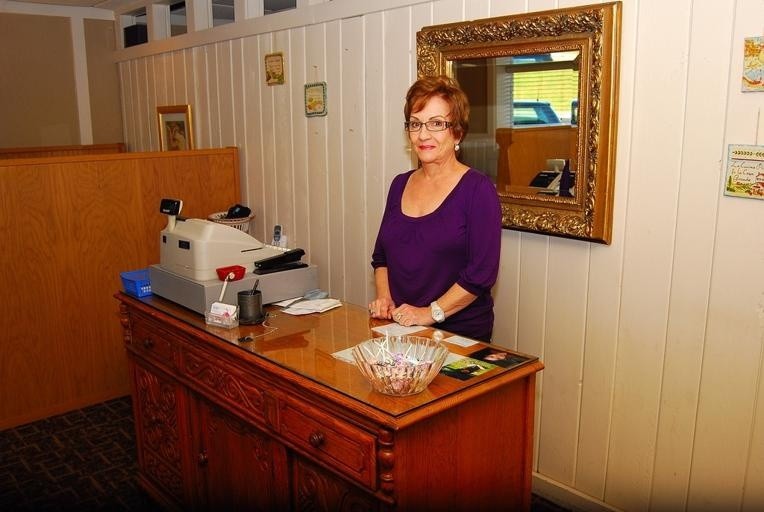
[
  {"x1": 207, "y1": 210, "x2": 255, "y2": 235},
  {"x1": 119, "y1": 269, "x2": 152, "y2": 298}
]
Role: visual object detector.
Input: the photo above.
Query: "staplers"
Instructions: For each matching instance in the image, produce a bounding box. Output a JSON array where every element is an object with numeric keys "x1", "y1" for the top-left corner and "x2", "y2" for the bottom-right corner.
[{"x1": 252, "y1": 248, "x2": 308, "y2": 276}]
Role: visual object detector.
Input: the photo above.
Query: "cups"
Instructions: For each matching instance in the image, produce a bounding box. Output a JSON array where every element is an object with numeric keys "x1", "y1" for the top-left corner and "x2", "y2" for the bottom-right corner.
[
  {"x1": 236, "y1": 290, "x2": 264, "y2": 325},
  {"x1": 239, "y1": 326, "x2": 264, "y2": 356}
]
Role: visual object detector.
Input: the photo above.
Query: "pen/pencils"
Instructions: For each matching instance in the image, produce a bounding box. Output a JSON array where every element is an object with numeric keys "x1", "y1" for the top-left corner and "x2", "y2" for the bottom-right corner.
[{"x1": 250, "y1": 278, "x2": 260, "y2": 294}]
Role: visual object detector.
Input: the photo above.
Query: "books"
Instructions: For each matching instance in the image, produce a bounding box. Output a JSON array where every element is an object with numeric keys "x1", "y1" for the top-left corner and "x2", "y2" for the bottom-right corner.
[{"x1": 528, "y1": 171, "x2": 562, "y2": 189}]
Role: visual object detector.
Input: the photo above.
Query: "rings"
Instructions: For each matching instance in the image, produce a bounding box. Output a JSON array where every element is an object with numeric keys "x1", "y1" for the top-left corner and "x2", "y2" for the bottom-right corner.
[{"x1": 395, "y1": 313, "x2": 402, "y2": 320}]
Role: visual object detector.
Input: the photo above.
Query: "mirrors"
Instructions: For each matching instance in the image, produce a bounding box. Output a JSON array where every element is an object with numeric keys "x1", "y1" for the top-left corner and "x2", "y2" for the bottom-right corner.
[{"x1": 414, "y1": 1, "x2": 625, "y2": 245}]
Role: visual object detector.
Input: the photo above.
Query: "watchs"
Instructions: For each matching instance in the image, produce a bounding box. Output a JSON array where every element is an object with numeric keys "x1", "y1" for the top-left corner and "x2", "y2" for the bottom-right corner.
[{"x1": 431, "y1": 300, "x2": 445, "y2": 323}]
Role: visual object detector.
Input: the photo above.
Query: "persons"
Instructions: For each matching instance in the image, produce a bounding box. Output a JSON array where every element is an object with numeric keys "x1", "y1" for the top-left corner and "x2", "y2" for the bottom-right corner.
[{"x1": 369, "y1": 75, "x2": 502, "y2": 344}]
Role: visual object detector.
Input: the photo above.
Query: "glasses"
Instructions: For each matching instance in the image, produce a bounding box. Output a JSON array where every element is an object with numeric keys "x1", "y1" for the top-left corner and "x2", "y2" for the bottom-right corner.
[{"x1": 404, "y1": 119, "x2": 461, "y2": 132}]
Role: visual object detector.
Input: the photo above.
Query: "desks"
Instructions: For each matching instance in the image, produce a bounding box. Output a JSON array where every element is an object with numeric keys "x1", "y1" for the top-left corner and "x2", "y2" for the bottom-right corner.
[{"x1": 111, "y1": 280, "x2": 545, "y2": 511}]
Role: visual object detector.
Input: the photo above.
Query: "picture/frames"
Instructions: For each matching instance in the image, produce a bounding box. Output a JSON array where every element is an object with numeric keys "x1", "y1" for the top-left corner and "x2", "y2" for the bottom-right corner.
[{"x1": 155, "y1": 103, "x2": 194, "y2": 153}]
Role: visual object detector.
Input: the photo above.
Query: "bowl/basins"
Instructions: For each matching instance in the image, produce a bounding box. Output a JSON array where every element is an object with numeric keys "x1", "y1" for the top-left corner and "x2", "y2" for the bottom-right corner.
[{"x1": 351, "y1": 336, "x2": 450, "y2": 395}]
